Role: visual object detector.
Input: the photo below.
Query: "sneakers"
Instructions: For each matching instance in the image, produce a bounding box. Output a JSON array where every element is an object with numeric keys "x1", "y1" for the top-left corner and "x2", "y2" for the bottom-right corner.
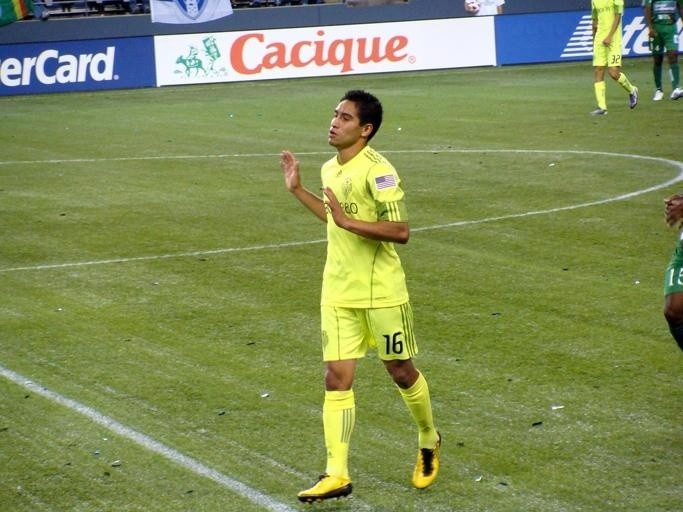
[
  {"x1": 670, "y1": 88, "x2": 683, "y2": 100},
  {"x1": 412, "y1": 431, "x2": 442, "y2": 489},
  {"x1": 629, "y1": 87, "x2": 638, "y2": 109},
  {"x1": 653, "y1": 91, "x2": 664, "y2": 101},
  {"x1": 590, "y1": 108, "x2": 608, "y2": 115},
  {"x1": 296, "y1": 473, "x2": 353, "y2": 503}
]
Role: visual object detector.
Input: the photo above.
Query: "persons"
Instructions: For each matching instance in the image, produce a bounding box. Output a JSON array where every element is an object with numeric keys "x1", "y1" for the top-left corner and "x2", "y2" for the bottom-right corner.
[
  {"x1": 281, "y1": 91, "x2": 440, "y2": 502},
  {"x1": 465, "y1": 0, "x2": 505, "y2": 16},
  {"x1": 641, "y1": 0, "x2": 683, "y2": 102},
  {"x1": 591, "y1": 0, "x2": 639, "y2": 116},
  {"x1": 664, "y1": 194, "x2": 683, "y2": 352}
]
